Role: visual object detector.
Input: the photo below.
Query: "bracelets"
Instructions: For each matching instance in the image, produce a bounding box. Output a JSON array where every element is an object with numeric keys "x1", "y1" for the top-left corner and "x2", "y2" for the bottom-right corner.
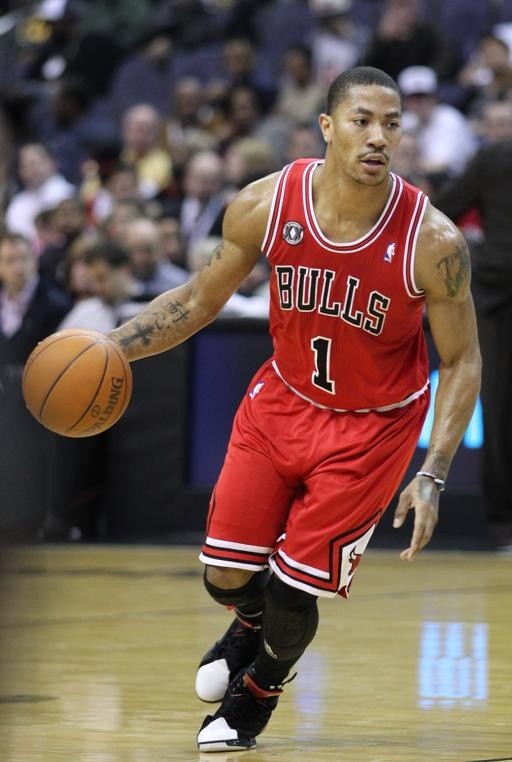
[{"x1": 417, "y1": 468, "x2": 447, "y2": 496}]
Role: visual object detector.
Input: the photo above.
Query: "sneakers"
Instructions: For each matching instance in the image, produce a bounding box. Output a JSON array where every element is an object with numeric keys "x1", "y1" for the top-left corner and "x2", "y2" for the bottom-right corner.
[
  {"x1": 195, "y1": 658, "x2": 285, "y2": 755},
  {"x1": 194, "y1": 614, "x2": 266, "y2": 706}
]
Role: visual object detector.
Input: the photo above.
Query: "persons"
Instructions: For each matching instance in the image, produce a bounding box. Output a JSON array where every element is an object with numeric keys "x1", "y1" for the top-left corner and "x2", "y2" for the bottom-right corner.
[
  {"x1": 435, "y1": 137, "x2": 509, "y2": 551},
  {"x1": 100, "y1": 66, "x2": 483, "y2": 756}
]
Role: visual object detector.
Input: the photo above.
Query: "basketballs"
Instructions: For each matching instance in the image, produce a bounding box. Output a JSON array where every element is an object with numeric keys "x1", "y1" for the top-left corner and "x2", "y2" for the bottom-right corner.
[{"x1": 21, "y1": 328, "x2": 134, "y2": 438}]
